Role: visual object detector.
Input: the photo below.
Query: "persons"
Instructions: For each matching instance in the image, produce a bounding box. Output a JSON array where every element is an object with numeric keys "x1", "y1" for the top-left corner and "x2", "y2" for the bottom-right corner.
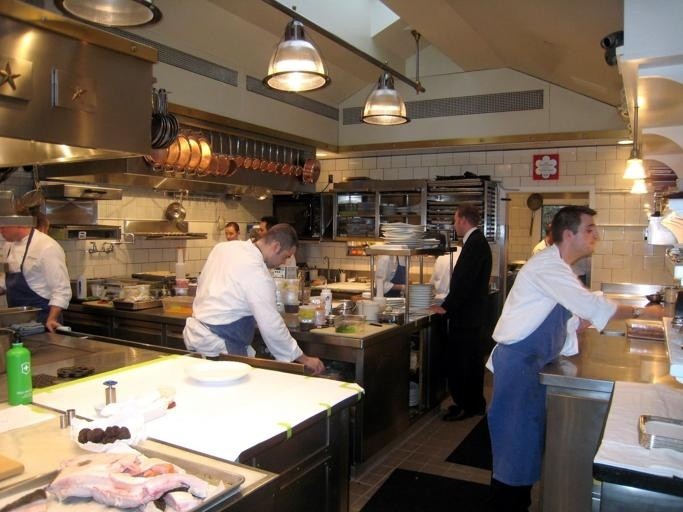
[
  {"x1": 429, "y1": 204, "x2": 494, "y2": 420},
  {"x1": 373, "y1": 254, "x2": 407, "y2": 296},
  {"x1": 180, "y1": 225, "x2": 326, "y2": 377},
  {"x1": 28, "y1": 211, "x2": 50, "y2": 235},
  {"x1": 427, "y1": 235, "x2": 461, "y2": 297},
  {"x1": 530, "y1": 223, "x2": 557, "y2": 254},
  {"x1": 223, "y1": 222, "x2": 240, "y2": 241},
  {"x1": 0, "y1": 223, "x2": 72, "y2": 333},
  {"x1": 479, "y1": 203, "x2": 663, "y2": 511},
  {"x1": 258, "y1": 216, "x2": 296, "y2": 267}
]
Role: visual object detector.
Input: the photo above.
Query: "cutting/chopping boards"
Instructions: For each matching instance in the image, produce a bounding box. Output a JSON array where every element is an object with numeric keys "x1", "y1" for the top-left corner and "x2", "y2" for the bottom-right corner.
[{"x1": 0, "y1": 454, "x2": 24, "y2": 480}]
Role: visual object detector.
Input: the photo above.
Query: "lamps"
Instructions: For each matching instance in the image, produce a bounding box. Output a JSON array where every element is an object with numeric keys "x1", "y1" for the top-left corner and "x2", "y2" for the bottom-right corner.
[
  {"x1": 622, "y1": 97, "x2": 649, "y2": 183},
  {"x1": 50, "y1": 1, "x2": 414, "y2": 128}
]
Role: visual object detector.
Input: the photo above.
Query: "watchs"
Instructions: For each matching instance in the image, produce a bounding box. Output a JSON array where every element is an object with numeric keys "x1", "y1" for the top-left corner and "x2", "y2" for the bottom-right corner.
[{"x1": 632, "y1": 305, "x2": 638, "y2": 317}]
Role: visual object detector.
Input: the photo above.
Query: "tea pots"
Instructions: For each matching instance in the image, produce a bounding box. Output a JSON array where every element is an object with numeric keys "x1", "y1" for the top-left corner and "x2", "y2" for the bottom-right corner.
[{"x1": 644, "y1": 292, "x2": 663, "y2": 303}]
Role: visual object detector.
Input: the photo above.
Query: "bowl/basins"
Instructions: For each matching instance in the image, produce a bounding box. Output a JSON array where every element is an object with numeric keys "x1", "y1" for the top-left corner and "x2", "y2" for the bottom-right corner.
[
  {"x1": 299, "y1": 291, "x2": 379, "y2": 332},
  {"x1": 71, "y1": 416, "x2": 149, "y2": 452},
  {"x1": 90, "y1": 284, "x2": 151, "y2": 298},
  {"x1": 175, "y1": 279, "x2": 196, "y2": 297}
]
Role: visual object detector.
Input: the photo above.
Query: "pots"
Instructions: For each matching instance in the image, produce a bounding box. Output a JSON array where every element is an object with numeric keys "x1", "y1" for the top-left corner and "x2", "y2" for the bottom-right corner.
[
  {"x1": 143, "y1": 121, "x2": 320, "y2": 185},
  {"x1": 163, "y1": 190, "x2": 189, "y2": 232},
  {"x1": 149, "y1": 88, "x2": 178, "y2": 150},
  {"x1": 525, "y1": 192, "x2": 543, "y2": 236}
]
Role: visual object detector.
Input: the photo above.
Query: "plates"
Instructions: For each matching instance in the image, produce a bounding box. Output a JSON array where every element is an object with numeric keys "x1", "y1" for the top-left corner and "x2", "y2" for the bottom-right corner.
[
  {"x1": 187, "y1": 359, "x2": 251, "y2": 385},
  {"x1": 379, "y1": 283, "x2": 433, "y2": 317},
  {"x1": 377, "y1": 221, "x2": 427, "y2": 248},
  {"x1": 380, "y1": 206, "x2": 410, "y2": 216}
]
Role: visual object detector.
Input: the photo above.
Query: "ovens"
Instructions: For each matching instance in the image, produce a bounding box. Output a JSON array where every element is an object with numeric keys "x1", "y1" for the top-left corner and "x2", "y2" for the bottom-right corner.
[{"x1": 271, "y1": 194, "x2": 322, "y2": 240}]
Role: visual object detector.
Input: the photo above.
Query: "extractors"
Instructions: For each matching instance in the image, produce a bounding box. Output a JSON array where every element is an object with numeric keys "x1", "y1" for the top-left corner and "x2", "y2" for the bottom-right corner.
[{"x1": 35, "y1": 179, "x2": 122, "y2": 241}]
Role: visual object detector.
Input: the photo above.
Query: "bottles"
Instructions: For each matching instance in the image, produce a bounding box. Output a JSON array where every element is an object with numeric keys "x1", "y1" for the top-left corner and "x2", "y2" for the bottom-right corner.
[
  {"x1": 4, "y1": 332, "x2": 32, "y2": 406},
  {"x1": 67, "y1": 408, "x2": 74, "y2": 427},
  {"x1": 321, "y1": 289, "x2": 331, "y2": 315},
  {"x1": 174, "y1": 248, "x2": 187, "y2": 278},
  {"x1": 58, "y1": 412, "x2": 67, "y2": 428},
  {"x1": 252, "y1": 221, "x2": 260, "y2": 228},
  {"x1": 345, "y1": 240, "x2": 370, "y2": 256},
  {"x1": 158, "y1": 278, "x2": 175, "y2": 296},
  {"x1": 75, "y1": 273, "x2": 87, "y2": 299},
  {"x1": 301, "y1": 286, "x2": 311, "y2": 304}
]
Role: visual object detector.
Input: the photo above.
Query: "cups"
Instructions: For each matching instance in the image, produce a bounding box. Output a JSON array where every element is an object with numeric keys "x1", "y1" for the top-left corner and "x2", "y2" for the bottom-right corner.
[
  {"x1": 246, "y1": 224, "x2": 252, "y2": 233},
  {"x1": 296, "y1": 269, "x2": 318, "y2": 282},
  {"x1": 663, "y1": 285, "x2": 677, "y2": 303},
  {"x1": 339, "y1": 273, "x2": 345, "y2": 281},
  {"x1": 94, "y1": 403, "x2": 105, "y2": 416},
  {"x1": 281, "y1": 277, "x2": 300, "y2": 312}
]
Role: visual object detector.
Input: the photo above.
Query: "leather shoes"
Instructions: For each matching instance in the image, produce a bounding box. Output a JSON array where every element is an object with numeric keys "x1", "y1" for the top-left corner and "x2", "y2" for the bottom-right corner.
[
  {"x1": 442, "y1": 405, "x2": 475, "y2": 421},
  {"x1": 448, "y1": 401, "x2": 486, "y2": 415}
]
